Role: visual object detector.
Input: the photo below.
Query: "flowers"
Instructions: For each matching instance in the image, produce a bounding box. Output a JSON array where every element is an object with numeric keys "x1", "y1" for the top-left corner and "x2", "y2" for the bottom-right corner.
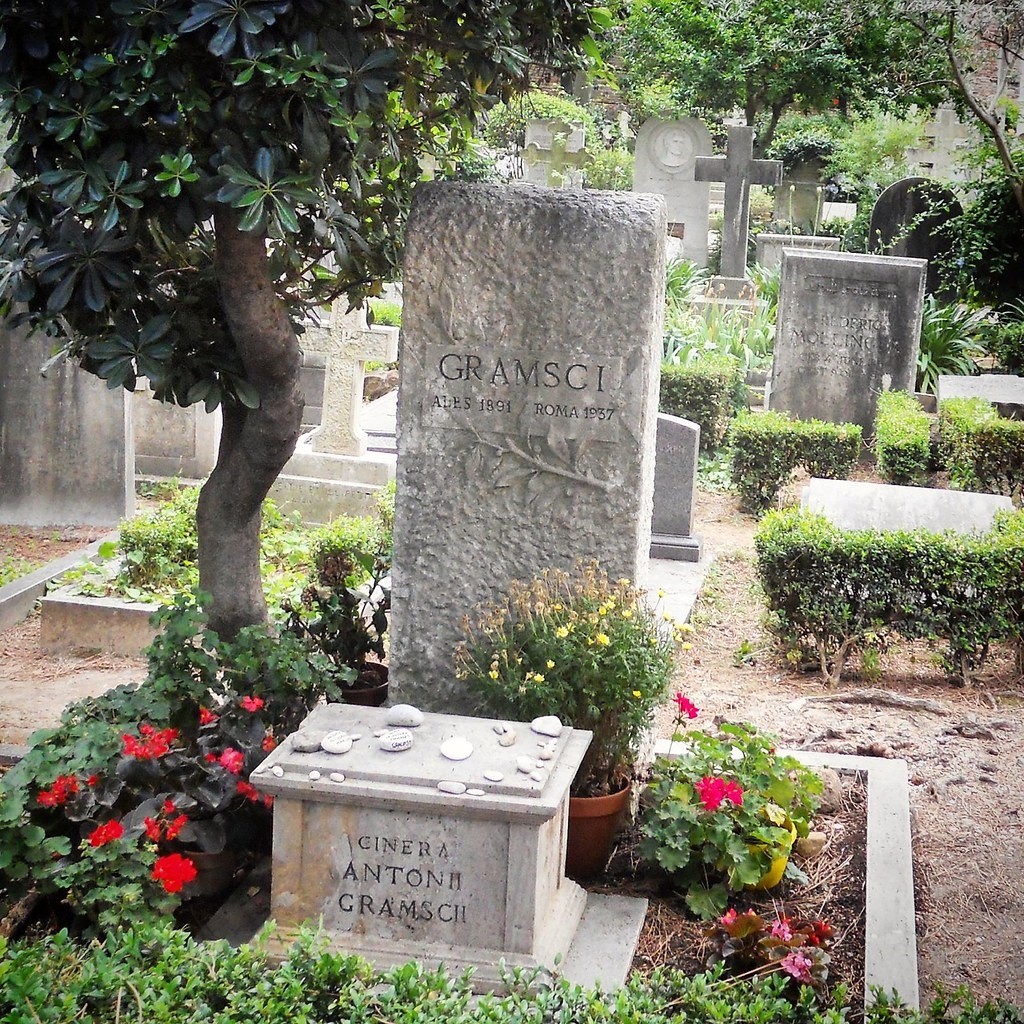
[
  {"x1": 640, "y1": 691, "x2": 822, "y2": 841},
  {"x1": 279, "y1": 539, "x2": 392, "y2": 668},
  {"x1": 457, "y1": 558, "x2": 692, "y2": 786},
  {"x1": 57, "y1": 724, "x2": 270, "y2": 846},
  {"x1": 565, "y1": 772, "x2": 632, "y2": 877}
]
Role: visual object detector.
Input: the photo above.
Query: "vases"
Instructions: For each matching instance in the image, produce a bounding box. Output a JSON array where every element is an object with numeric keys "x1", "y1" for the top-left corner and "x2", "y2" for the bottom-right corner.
[
  {"x1": 729, "y1": 809, "x2": 800, "y2": 890},
  {"x1": 323, "y1": 657, "x2": 389, "y2": 706},
  {"x1": 182, "y1": 843, "x2": 236, "y2": 897}
]
